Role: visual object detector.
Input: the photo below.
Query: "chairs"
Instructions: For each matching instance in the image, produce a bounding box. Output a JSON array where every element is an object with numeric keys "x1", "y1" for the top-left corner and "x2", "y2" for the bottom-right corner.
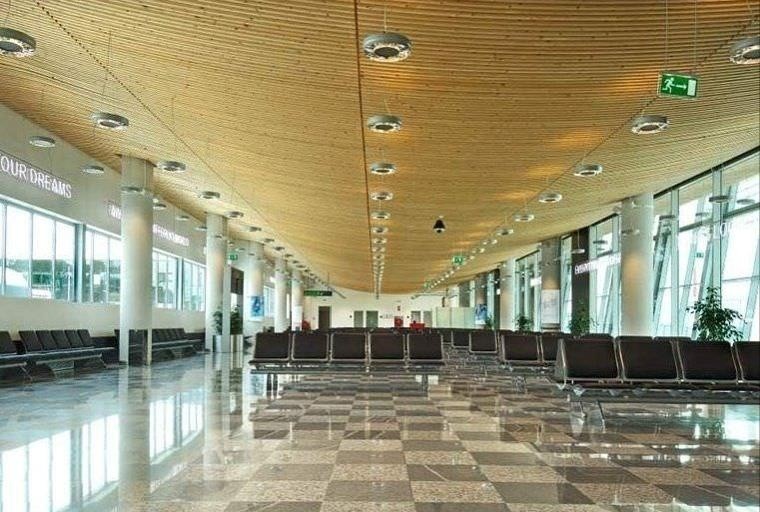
[{"x1": 0, "y1": 327, "x2": 208, "y2": 383}]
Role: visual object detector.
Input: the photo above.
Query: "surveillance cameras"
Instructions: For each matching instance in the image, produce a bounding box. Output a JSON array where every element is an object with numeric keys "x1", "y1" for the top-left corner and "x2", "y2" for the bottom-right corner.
[{"x1": 432, "y1": 220, "x2": 446, "y2": 234}]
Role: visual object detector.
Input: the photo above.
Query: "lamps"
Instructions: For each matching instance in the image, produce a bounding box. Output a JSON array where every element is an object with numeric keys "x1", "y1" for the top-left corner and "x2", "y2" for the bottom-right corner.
[{"x1": 0, "y1": 8, "x2": 759, "y2": 302}]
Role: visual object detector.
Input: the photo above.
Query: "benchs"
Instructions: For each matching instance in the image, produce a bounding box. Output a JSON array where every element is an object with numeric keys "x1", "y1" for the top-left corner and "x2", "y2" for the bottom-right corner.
[
  {"x1": 249, "y1": 329, "x2": 689, "y2": 387},
  {"x1": 547, "y1": 340, "x2": 759, "y2": 420}
]
